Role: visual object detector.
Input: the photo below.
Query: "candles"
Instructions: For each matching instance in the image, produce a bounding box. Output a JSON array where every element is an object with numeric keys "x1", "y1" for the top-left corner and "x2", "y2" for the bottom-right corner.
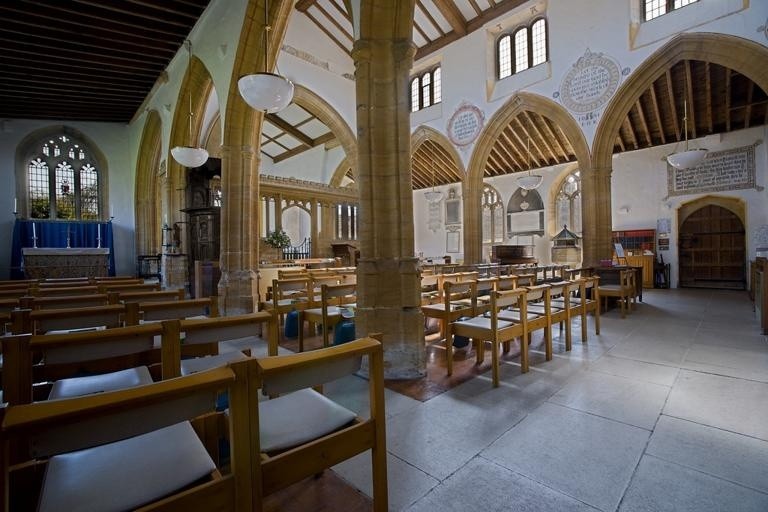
[
  {"x1": 97, "y1": 224, "x2": 101, "y2": 238},
  {"x1": 32, "y1": 222, "x2": 36, "y2": 238}
]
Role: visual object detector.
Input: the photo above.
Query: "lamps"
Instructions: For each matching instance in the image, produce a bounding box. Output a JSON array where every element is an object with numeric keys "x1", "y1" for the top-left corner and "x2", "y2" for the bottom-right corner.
[
  {"x1": 423, "y1": 143, "x2": 443, "y2": 204},
  {"x1": 665, "y1": 58, "x2": 710, "y2": 173},
  {"x1": 515, "y1": 113, "x2": 543, "y2": 190},
  {"x1": 235, "y1": 1, "x2": 296, "y2": 116},
  {"x1": 171, "y1": 41, "x2": 210, "y2": 169}
]
